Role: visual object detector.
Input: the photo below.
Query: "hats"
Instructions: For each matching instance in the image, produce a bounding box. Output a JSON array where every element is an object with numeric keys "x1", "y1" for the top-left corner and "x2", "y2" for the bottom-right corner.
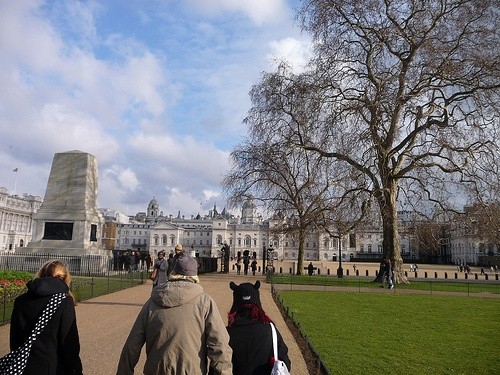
[
  {"x1": 229, "y1": 280, "x2": 261, "y2": 313},
  {"x1": 174, "y1": 256, "x2": 198, "y2": 276},
  {"x1": 175, "y1": 244, "x2": 182, "y2": 250}
]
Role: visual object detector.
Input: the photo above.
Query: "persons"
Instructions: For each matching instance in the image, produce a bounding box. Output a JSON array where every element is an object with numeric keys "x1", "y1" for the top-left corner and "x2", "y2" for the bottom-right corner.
[
  {"x1": 409, "y1": 264, "x2": 498, "y2": 275},
  {"x1": 380, "y1": 257, "x2": 394, "y2": 290},
  {"x1": 117, "y1": 253, "x2": 233, "y2": 375},
  {"x1": 308, "y1": 262, "x2": 313, "y2": 276},
  {"x1": 227, "y1": 281, "x2": 291, "y2": 375},
  {"x1": 235, "y1": 257, "x2": 258, "y2": 276},
  {"x1": 10, "y1": 260, "x2": 83, "y2": 375},
  {"x1": 117, "y1": 244, "x2": 183, "y2": 291}
]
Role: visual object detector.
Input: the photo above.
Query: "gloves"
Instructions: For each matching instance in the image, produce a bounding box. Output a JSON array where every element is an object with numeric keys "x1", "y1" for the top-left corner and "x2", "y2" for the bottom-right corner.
[{"x1": 156, "y1": 265, "x2": 160, "y2": 268}]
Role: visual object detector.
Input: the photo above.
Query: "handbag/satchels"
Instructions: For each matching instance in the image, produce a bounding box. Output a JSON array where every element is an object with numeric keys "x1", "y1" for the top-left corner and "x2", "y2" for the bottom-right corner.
[
  {"x1": 238, "y1": 261, "x2": 241, "y2": 265},
  {"x1": 252, "y1": 262, "x2": 253, "y2": 266},
  {"x1": 150, "y1": 268, "x2": 158, "y2": 281},
  {"x1": 271, "y1": 361, "x2": 290, "y2": 375},
  {"x1": 0, "y1": 347, "x2": 28, "y2": 375}
]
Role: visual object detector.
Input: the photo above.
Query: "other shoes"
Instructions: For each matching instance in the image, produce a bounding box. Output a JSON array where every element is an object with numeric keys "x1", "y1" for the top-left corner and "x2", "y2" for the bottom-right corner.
[
  {"x1": 379, "y1": 286, "x2": 384, "y2": 288},
  {"x1": 391, "y1": 285, "x2": 394, "y2": 289},
  {"x1": 389, "y1": 285, "x2": 392, "y2": 289}
]
